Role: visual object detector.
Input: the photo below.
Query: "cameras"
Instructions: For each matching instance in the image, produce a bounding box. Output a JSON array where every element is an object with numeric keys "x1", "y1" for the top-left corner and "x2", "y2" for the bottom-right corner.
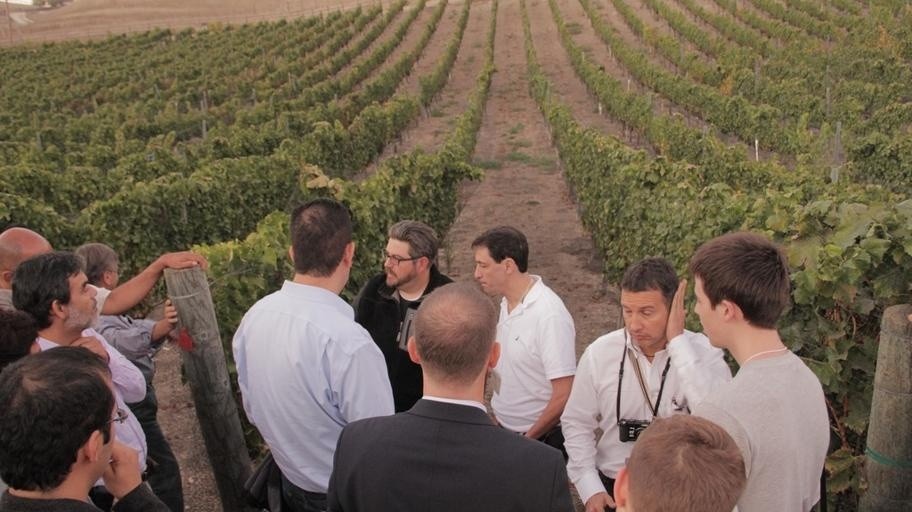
[{"x1": 618, "y1": 419, "x2": 651, "y2": 443}]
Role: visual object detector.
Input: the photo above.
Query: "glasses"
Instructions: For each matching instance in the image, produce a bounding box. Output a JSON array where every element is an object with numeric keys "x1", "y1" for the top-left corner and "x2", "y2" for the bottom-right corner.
[
  {"x1": 100, "y1": 408, "x2": 129, "y2": 425},
  {"x1": 382, "y1": 249, "x2": 426, "y2": 265}
]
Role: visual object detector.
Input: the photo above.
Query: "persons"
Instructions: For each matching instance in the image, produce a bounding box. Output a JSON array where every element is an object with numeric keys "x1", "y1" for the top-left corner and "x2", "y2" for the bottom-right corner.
[
  {"x1": 231, "y1": 197, "x2": 401, "y2": 511},
  {"x1": 327, "y1": 278, "x2": 575, "y2": 512},
  {"x1": 470, "y1": 223, "x2": 573, "y2": 466},
  {"x1": 612, "y1": 412, "x2": 746, "y2": 512},
  {"x1": 72, "y1": 241, "x2": 187, "y2": 511},
  {"x1": 348, "y1": 217, "x2": 459, "y2": 413},
  {"x1": 11, "y1": 249, "x2": 154, "y2": 512},
  {"x1": 1, "y1": 225, "x2": 206, "y2": 318},
  {"x1": 557, "y1": 257, "x2": 733, "y2": 512},
  {"x1": 2, "y1": 302, "x2": 41, "y2": 375},
  {"x1": 1, "y1": 344, "x2": 172, "y2": 512},
  {"x1": 689, "y1": 231, "x2": 831, "y2": 512}
]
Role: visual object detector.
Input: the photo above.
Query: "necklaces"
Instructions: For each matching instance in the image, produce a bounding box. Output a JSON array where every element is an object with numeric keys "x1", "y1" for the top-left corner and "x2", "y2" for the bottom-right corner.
[
  {"x1": 643, "y1": 352, "x2": 654, "y2": 358},
  {"x1": 741, "y1": 346, "x2": 788, "y2": 363}
]
